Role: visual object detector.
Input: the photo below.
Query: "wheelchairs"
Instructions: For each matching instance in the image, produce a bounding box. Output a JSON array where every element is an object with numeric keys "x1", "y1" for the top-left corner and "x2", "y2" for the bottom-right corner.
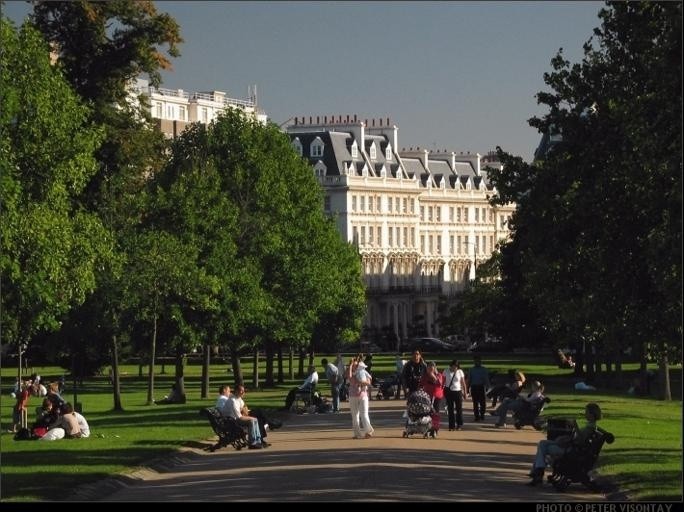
[{"x1": 293, "y1": 383, "x2": 318, "y2": 416}]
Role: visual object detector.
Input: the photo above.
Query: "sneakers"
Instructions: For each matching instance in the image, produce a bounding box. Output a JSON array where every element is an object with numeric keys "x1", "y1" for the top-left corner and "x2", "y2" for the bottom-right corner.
[
  {"x1": 474, "y1": 415, "x2": 485, "y2": 423},
  {"x1": 351, "y1": 432, "x2": 363, "y2": 439},
  {"x1": 248, "y1": 440, "x2": 268, "y2": 449},
  {"x1": 447, "y1": 425, "x2": 463, "y2": 431},
  {"x1": 488, "y1": 410, "x2": 505, "y2": 428},
  {"x1": 364, "y1": 428, "x2": 374, "y2": 439}
]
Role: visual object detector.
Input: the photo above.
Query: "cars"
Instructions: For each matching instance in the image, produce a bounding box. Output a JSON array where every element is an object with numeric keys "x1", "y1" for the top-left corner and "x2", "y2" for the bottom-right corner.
[{"x1": 359, "y1": 336, "x2": 506, "y2": 352}]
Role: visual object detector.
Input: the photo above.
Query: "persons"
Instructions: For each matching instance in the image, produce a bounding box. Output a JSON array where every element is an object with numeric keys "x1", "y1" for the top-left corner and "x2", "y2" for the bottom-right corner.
[
  {"x1": 465, "y1": 355, "x2": 489, "y2": 421},
  {"x1": 11, "y1": 371, "x2": 64, "y2": 397},
  {"x1": 217, "y1": 384, "x2": 283, "y2": 448},
  {"x1": 308, "y1": 351, "x2": 314, "y2": 374},
  {"x1": 565, "y1": 355, "x2": 574, "y2": 368},
  {"x1": 487, "y1": 379, "x2": 545, "y2": 430},
  {"x1": 220, "y1": 384, "x2": 263, "y2": 451},
  {"x1": 151, "y1": 384, "x2": 175, "y2": 406},
  {"x1": 441, "y1": 359, "x2": 467, "y2": 431},
  {"x1": 487, "y1": 371, "x2": 526, "y2": 410},
  {"x1": 523, "y1": 402, "x2": 602, "y2": 489},
  {"x1": 7, "y1": 387, "x2": 31, "y2": 434},
  {"x1": 32, "y1": 398, "x2": 91, "y2": 440},
  {"x1": 278, "y1": 365, "x2": 320, "y2": 412},
  {"x1": 334, "y1": 351, "x2": 444, "y2": 440},
  {"x1": 320, "y1": 357, "x2": 344, "y2": 413}
]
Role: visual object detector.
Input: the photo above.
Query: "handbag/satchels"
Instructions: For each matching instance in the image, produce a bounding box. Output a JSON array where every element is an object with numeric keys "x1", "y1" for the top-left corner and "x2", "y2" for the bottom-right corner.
[{"x1": 444, "y1": 386, "x2": 452, "y2": 401}]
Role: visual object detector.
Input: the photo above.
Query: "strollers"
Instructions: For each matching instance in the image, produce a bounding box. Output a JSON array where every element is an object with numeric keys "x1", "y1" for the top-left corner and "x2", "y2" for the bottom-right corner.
[
  {"x1": 377, "y1": 373, "x2": 403, "y2": 401},
  {"x1": 401, "y1": 387, "x2": 436, "y2": 439}
]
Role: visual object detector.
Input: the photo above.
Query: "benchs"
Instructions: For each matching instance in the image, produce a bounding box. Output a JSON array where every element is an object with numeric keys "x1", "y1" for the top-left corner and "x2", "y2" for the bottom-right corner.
[
  {"x1": 497, "y1": 381, "x2": 527, "y2": 403},
  {"x1": 200, "y1": 406, "x2": 250, "y2": 450},
  {"x1": 511, "y1": 396, "x2": 551, "y2": 430},
  {"x1": 546, "y1": 427, "x2": 615, "y2": 491}
]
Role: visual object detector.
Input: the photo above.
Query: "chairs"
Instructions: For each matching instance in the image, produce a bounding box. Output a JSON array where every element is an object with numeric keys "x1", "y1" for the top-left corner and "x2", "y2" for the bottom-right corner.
[{"x1": 293, "y1": 383, "x2": 316, "y2": 411}]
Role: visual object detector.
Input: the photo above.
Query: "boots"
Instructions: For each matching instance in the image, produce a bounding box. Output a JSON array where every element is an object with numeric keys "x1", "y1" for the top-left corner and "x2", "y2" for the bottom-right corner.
[{"x1": 527, "y1": 467, "x2": 544, "y2": 487}]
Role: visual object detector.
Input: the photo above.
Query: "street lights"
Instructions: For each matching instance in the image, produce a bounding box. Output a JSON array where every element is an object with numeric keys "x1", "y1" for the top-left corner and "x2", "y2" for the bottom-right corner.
[
  {"x1": 354, "y1": 229, "x2": 374, "y2": 254},
  {"x1": 463, "y1": 241, "x2": 477, "y2": 275}
]
